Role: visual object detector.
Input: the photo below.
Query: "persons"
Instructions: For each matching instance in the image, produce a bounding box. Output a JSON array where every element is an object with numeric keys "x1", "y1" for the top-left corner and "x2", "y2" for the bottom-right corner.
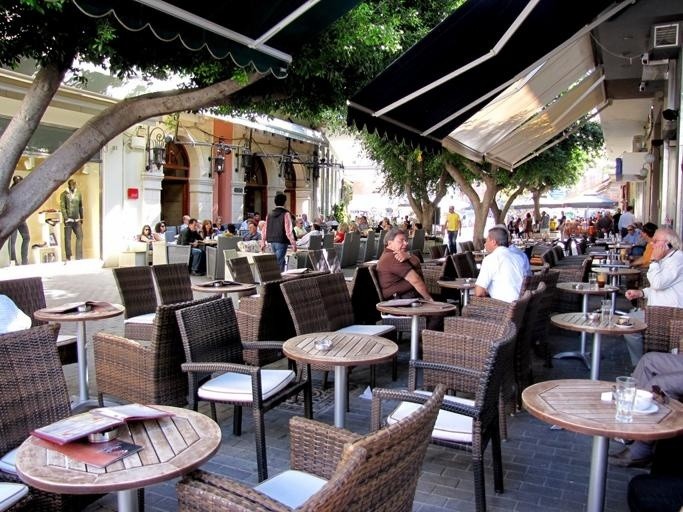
[
  {"x1": 375, "y1": 228, "x2": 448, "y2": 349},
  {"x1": 444, "y1": 205, "x2": 461, "y2": 256},
  {"x1": 474, "y1": 223, "x2": 531, "y2": 303},
  {"x1": 508, "y1": 207, "x2": 637, "y2": 238},
  {"x1": 60, "y1": 179, "x2": 84, "y2": 261},
  {"x1": 8, "y1": 175, "x2": 30, "y2": 266},
  {"x1": 138, "y1": 194, "x2": 413, "y2": 276},
  {"x1": 625, "y1": 221, "x2": 682, "y2": 392}
]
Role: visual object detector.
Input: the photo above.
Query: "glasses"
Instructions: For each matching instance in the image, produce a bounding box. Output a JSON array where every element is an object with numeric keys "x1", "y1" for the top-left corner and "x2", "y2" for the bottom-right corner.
[{"x1": 652, "y1": 385, "x2": 668, "y2": 405}]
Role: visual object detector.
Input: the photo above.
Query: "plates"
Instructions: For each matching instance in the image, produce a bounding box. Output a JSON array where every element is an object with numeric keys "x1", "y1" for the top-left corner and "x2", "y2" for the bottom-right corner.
[
  {"x1": 614, "y1": 323, "x2": 633, "y2": 329},
  {"x1": 633, "y1": 403, "x2": 660, "y2": 414}
]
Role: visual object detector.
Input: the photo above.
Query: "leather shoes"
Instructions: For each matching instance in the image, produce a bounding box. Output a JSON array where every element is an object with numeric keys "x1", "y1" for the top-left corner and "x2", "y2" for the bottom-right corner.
[{"x1": 607, "y1": 449, "x2": 653, "y2": 467}]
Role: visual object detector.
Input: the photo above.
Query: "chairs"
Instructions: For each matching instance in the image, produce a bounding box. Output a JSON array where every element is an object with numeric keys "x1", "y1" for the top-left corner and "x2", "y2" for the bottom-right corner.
[
  {"x1": 0, "y1": 324, "x2": 110, "y2": 511},
  {"x1": 370, "y1": 320, "x2": 519, "y2": 511},
  {"x1": 644, "y1": 303, "x2": 682, "y2": 353},
  {"x1": 416, "y1": 289, "x2": 532, "y2": 442},
  {"x1": 172, "y1": 296, "x2": 296, "y2": 483},
  {"x1": 175, "y1": 382, "x2": 448, "y2": 512},
  {"x1": 16, "y1": 403, "x2": 224, "y2": 511},
  {"x1": 0, "y1": 277, "x2": 89, "y2": 367},
  {"x1": 111, "y1": 265, "x2": 161, "y2": 342},
  {"x1": 281, "y1": 272, "x2": 401, "y2": 392},
  {"x1": 112, "y1": 215, "x2": 683, "y2": 319},
  {"x1": 91, "y1": 293, "x2": 225, "y2": 408}
]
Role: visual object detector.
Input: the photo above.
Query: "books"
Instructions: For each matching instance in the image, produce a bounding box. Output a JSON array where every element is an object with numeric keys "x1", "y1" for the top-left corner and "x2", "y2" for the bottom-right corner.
[
  {"x1": 378, "y1": 297, "x2": 430, "y2": 307},
  {"x1": 436, "y1": 278, "x2": 477, "y2": 286},
  {"x1": 38, "y1": 300, "x2": 112, "y2": 314},
  {"x1": 27, "y1": 438, "x2": 145, "y2": 469},
  {"x1": 29, "y1": 402, "x2": 176, "y2": 446},
  {"x1": 196, "y1": 279, "x2": 242, "y2": 287}
]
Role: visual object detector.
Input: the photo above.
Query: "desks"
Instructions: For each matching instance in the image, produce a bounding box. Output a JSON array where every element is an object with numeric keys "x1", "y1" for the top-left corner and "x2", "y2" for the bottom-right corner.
[
  {"x1": 373, "y1": 295, "x2": 458, "y2": 389},
  {"x1": 549, "y1": 311, "x2": 648, "y2": 448},
  {"x1": 33, "y1": 301, "x2": 127, "y2": 416},
  {"x1": 282, "y1": 330, "x2": 400, "y2": 429},
  {"x1": 514, "y1": 378, "x2": 683, "y2": 512}
]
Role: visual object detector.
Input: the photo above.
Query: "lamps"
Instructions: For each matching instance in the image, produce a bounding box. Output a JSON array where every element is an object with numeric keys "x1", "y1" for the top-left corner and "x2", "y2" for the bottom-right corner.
[
  {"x1": 235, "y1": 133, "x2": 254, "y2": 174},
  {"x1": 206, "y1": 137, "x2": 226, "y2": 180},
  {"x1": 144, "y1": 122, "x2": 165, "y2": 173},
  {"x1": 638, "y1": 159, "x2": 648, "y2": 179},
  {"x1": 278, "y1": 155, "x2": 291, "y2": 179},
  {"x1": 644, "y1": 142, "x2": 656, "y2": 162}
]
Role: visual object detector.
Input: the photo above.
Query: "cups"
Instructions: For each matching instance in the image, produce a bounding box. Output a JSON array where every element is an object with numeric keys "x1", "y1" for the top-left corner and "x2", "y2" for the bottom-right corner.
[
  {"x1": 631, "y1": 387, "x2": 655, "y2": 410},
  {"x1": 614, "y1": 375, "x2": 638, "y2": 423},
  {"x1": 600, "y1": 299, "x2": 612, "y2": 326},
  {"x1": 597, "y1": 272, "x2": 606, "y2": 289},
  {"x1": 587, "y1": 272, "x2": 597, "y2": 290}
]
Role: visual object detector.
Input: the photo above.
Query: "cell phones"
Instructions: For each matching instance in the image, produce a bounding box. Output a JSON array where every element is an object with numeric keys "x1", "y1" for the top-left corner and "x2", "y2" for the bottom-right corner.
[{"x1": 665, "y1": 241, "x2": 673, "y2": 251}]
